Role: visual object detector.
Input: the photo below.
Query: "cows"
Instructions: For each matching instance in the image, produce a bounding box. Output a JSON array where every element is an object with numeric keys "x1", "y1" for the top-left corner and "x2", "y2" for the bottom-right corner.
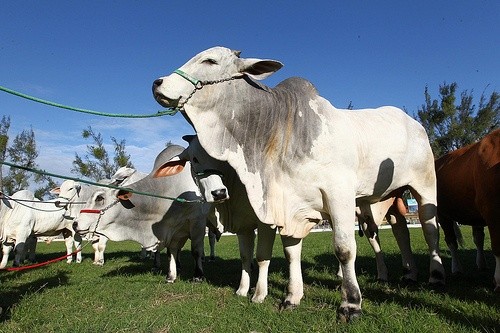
[
  {"x1": 435, "y1": 127, "x2": 500, "y2": 293},
  {"x1": 6, "y1": 189, "x2": 84, "y2": 267},
  {"x1": 178, "y1": 133, "x2": 419, "y2": 304},
  {"x1": 49, "y1": 177, "x2": 157, "y2": 267},
  {"x1": 151, "y1": 45, "x2": 447, "y2": 326},
  {"x1": 71, "y1": 144, "x2": 224, "y2": 285}
]
